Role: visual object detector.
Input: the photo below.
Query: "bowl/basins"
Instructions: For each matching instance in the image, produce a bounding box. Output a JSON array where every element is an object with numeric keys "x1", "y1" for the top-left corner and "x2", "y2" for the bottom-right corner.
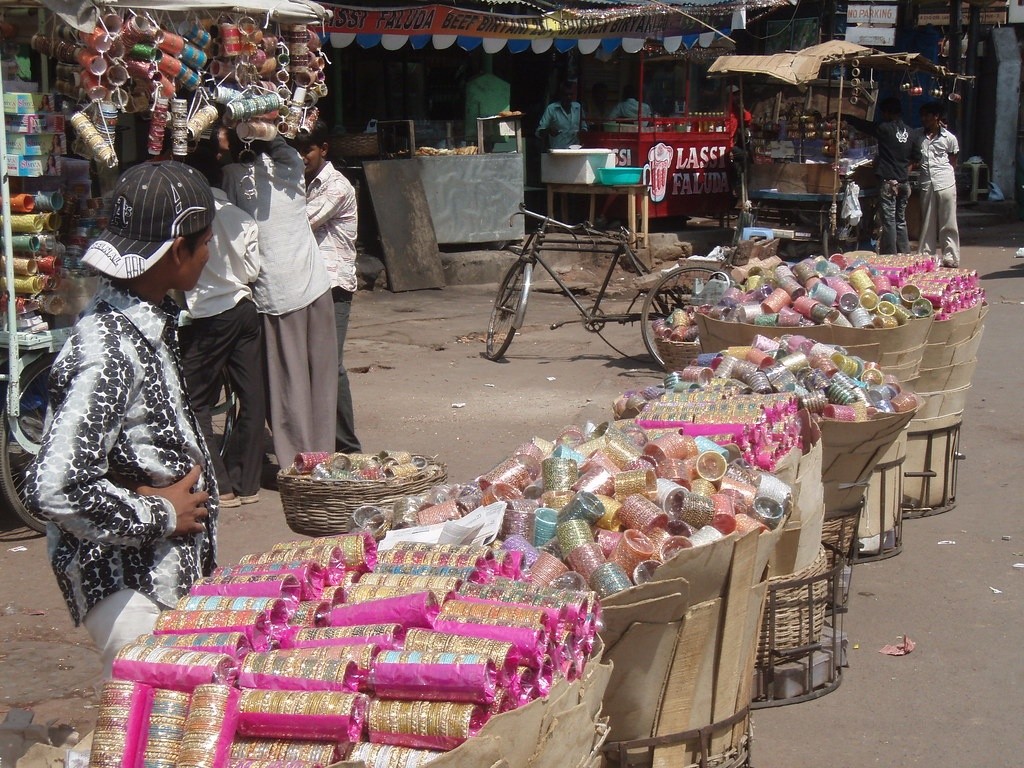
[
  {"x1": 598, "y1": 168, "x2": 643, "y2": 186},
  {"x1": 432, "y1": 121, "x2": 464, "y2": 137}
]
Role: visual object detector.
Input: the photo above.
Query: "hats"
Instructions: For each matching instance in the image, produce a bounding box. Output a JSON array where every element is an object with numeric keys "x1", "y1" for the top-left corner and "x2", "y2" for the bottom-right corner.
[
  {"x1": 726, "y1": 84, "x2": 740, "y2": 95},
  {"x1": 82, "y1": 160, "x2": 216, "y2": 279}
]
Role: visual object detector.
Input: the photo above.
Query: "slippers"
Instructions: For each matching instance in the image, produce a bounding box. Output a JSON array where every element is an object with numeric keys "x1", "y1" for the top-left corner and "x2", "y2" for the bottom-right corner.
[{"x1": 218, "y1": 492, "x2": 259, "y2": 509}]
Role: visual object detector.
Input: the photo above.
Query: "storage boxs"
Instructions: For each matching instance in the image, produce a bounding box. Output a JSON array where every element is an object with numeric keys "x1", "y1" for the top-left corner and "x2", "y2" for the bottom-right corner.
[
  {"x1": 540, "y1": 154, "x2": 616, "y2": 184},
  {"x1": 1, "y1": 80, "x2": 94, "y2": 214}
]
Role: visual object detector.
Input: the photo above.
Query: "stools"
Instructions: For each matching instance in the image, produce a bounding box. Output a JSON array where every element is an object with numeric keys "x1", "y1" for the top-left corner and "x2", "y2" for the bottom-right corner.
[
  {"x1": 743, "y1": 227, "x2": 774, "y2": 243},
  {"x1": 959, "y1": 164, "x2": 991, "y2": 201}
]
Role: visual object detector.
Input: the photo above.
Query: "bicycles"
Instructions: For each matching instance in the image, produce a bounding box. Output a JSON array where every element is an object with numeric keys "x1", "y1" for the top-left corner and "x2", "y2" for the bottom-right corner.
[{"x1": 484, "y1": 200, "x2": 737, "y2": 371}]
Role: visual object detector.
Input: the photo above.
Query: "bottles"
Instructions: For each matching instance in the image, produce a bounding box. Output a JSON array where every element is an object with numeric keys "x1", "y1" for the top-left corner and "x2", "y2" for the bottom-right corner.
[
  {"x1": 686, "y1": 112, "x2": 692, "y2": 132},
  {"x1": 703, "y1": 112, "x2": 713, "y2": 132},
  {"x1": 677, "y1": 123, "x2": 686, "y2": 132},
  {"x1": 699, "y1": 113, "x2": 703, "y2": 133},
  {"x1": 693, "y1": 112, "x2": 699, "y2": 132},
  {"x1": 712, "y1": 112, "x2": 726, "y2": 133},
  {"x1": 647, "y1": 113, "x2": 662, "y2": 127},
  {"x1": 460, "y1": 136, "x2": 478, "y2": 147}
]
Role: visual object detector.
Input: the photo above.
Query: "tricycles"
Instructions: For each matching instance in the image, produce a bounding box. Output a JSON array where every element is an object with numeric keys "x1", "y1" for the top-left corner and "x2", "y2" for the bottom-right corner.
[
  {"x1": 1, "y1": 335, "x2": 237, "y2": 536},
  {"x1": 733, "y1": 191, "x2": 877, "y2": 260}
]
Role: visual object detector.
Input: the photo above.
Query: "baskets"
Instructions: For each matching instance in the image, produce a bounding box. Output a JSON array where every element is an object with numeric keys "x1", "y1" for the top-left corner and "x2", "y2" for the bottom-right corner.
[
  {"x1": 753, "y1": 540, "x2": 829, "y2": 669},
  {"x1": 822, "y1": 513, "x2": 859, "y2": 605},
  {"x1": 276, "y1": 451, "x2": 447, "y2": 534},
  {"x1": 653, "y1": 337, "x2": 702, "y2": 374}
]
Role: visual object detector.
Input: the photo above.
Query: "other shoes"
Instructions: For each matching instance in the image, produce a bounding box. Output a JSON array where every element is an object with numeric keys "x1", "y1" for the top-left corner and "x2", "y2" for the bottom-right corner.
[
  {"x1": 596, "y1": 213, "x2": 608, "y2": 224},
  {"x1": 945, "y1": 260, "x2": 958, "y2": 268}
]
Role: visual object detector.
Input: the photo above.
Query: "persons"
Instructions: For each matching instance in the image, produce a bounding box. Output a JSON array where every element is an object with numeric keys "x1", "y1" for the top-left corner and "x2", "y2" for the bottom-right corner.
[
  {"x1": 286, "y1": 119, "x2": 363, "y2": 454},
  {"x1": 824, "y1": 97, "x2": 922, "y2": 254},
  {"x1": 173, "y1": 158, "x2": 268, "y2": 508},
  {"x1": 912, "y1": 102, "x2": 960, "y2": 269},
  {"x1": 24, "y1": 161, "x2": 219, "y2": 683},
  {"x1": 533, "y1": 82, "x2": 592, "y2": 227},
  {"x1": 604, "y1": 84, "x2": 652, "y2": 128},
  {"x1": 715, "y1": 84, "x2": 750, "y2": 124},
  {"x1": 221, "y1": 132, "x2": 339, "y2": 469}
]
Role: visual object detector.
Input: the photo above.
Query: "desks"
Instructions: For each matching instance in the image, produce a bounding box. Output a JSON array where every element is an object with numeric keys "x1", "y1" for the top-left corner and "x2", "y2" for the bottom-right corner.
[{"x1": 547, "y1": 184, "x2": 649, "y2": 249}]
